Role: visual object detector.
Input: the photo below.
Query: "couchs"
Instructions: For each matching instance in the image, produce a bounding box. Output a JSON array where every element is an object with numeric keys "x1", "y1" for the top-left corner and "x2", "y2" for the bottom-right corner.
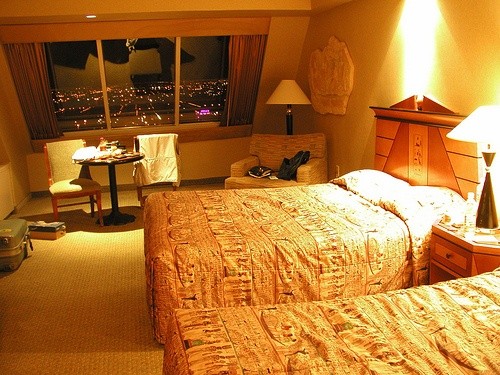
[{"x1": 225, "y1": 133, "x2": 329, "y2": 189}]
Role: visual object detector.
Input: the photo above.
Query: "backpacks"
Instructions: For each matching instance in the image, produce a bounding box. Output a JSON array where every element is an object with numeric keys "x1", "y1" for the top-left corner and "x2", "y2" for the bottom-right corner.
[{"x1": 278, "y1": 150, "x2": 310, "y2": 181}]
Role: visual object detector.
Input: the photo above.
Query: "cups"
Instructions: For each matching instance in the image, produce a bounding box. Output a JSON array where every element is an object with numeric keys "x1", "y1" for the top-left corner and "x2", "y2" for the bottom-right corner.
[{"x1": 111, "y1": 146, "x2": 117, "y2": 152}]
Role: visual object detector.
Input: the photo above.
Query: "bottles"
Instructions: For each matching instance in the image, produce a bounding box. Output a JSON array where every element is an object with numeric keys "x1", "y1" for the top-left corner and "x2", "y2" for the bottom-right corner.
[
  {"x1": 100, "y1": 138, "x2": 107, "y2": 160},
  {"x1": 465, "y1": 192, "x2": 478, "y2": 228}
]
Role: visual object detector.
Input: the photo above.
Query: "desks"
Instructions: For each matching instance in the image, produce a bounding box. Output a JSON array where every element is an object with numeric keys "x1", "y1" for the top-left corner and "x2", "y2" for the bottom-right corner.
[{"x1": 71, "y1": 150, "x2": 145, "y2": 232}]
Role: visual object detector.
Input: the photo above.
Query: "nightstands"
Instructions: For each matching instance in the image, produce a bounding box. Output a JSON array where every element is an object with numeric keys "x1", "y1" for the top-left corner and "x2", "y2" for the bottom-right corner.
[{"x1": 429, "y1": 223, "x2": 500, "y2": 283}]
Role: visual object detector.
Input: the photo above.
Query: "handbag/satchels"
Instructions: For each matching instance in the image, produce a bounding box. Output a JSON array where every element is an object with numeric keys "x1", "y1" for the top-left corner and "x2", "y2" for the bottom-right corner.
[{"x1": 247, "y1": 166, "x2": 272, "y2": 178}]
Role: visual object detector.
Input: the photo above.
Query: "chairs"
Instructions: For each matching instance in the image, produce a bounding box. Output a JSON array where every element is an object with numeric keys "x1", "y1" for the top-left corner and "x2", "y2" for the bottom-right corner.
[
  {"x1": 130, "y1": 134, "x2": 183, "y2": 207},
  {"x1": 42, "y1": 139, "x2": 103, "y2": 231}
]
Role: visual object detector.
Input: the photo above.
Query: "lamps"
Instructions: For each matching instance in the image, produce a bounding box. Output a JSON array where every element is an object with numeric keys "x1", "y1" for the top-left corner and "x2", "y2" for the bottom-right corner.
[
  {"x1": 446, "y1": 104, "x2": 500, "y2": 229},
  {"x1": 266, "y1": 79, "x2": 312, "y2": 134}
]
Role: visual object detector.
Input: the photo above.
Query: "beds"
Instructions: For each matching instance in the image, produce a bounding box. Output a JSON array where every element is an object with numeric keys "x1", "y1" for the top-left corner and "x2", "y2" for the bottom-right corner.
[
  {"x1": 161, "y1": 268, "x2": 500, "y2": 375},
  {"x1": 143, "y1": 94, "x2": 484, "y2": 346}
]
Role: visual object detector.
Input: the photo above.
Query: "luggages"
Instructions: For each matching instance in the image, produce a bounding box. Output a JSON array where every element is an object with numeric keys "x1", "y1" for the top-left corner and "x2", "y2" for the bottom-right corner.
[{"x1": 0, "y1": 218, "x2": 33, "y2": 272}]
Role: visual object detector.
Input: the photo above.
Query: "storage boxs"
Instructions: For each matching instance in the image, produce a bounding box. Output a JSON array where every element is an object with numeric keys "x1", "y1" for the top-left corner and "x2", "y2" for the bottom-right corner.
[{"x1": 26, "y1": 220, "x2": 67, "y2": 240}]
